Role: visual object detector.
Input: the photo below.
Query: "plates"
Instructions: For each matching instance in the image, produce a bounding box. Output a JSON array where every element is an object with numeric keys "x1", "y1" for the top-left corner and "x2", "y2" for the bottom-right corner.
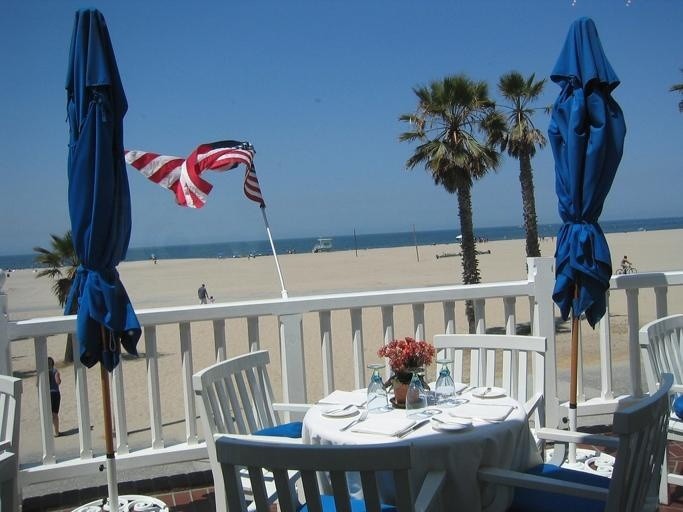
[
  {"x1": 472, "y1": 386, "x2": 504, "y2": 397},
  {"x1": 428, "y1": 381, "x2": 466, "y2": 393},
  {"x1": 320, "y1": 404, "x2": 358, "y2": 416},
  {"x1": 432, "y1": 418, "x2": 472, "y2": 431}
]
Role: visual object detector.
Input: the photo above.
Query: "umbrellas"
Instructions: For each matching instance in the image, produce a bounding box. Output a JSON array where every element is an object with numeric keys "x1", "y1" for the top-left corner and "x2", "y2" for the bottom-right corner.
[
  {"x1": 456, "y1": 234, "x2": 462, "y2": 239},
  {"x1": 63, "y1": 9, "x2": 145, "y2": 512},
  {"x1": 549, "y1": 16, "x2": 626, "y2": 463}
]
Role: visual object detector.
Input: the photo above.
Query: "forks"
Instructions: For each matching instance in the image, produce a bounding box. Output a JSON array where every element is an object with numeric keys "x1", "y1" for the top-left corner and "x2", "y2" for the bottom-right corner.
[{"x1": 338, "y1": 410, "x2": 368, "y2": 432}]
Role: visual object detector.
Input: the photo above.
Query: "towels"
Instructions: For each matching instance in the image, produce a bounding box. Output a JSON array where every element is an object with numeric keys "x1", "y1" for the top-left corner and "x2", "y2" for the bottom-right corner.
[
  {"x1": 352, "y1": 416, "x2": 416, "y2": 436},
  {"x1": 448, "y1": 402, "x2": 514, "y2": 423},
  {"x1": 319, "y1": 389, "x2": 373, "y2": 408}
]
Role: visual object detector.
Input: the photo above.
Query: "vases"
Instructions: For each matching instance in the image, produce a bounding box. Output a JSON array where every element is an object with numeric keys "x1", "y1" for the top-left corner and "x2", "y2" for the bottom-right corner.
[{"x1": 390, "y1": 377, "x2": 424, "y2": 409}]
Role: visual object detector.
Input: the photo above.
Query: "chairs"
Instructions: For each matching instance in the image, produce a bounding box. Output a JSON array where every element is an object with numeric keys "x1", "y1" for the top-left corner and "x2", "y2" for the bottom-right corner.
[
  {"x1": 435, "y1": 335, "x2": 547, "y2": 433},
  {"x1": 638, "y1": 314, "x2": 683, "y2": 505},
  {"x1": 477, "y1": 371, "x2": 674, "y2": 511},
  {"x1": 216, "y1": 438, "x2": 447, "y2": 511},
  {"x1": 0, "y1": 375, "x2": 23, "y2": 512},
  {"x1": 191, "y1": 351, "x2": 315, "y2": 509}
]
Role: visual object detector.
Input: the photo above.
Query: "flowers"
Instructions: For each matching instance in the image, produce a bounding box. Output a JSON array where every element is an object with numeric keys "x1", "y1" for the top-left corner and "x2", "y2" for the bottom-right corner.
[{"x1": 377, "y1": 336, "x2": 435, "y2": 392}]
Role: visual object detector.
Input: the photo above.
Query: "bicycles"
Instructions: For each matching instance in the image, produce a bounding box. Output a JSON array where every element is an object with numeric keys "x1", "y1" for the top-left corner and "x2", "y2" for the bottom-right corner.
[{"x1": 615, "y1": 263, "x2": 638, "y2": 275}]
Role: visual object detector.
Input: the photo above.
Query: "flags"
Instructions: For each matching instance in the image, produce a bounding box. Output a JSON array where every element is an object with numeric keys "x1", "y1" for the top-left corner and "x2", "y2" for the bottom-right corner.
[{"x1": 122, "y1": 139, "x2": 264, "y2": 210}]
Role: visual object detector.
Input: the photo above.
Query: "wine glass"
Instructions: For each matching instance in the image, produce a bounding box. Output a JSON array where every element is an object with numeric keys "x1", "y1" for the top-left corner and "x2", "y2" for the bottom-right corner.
[
  {"x1": 435, "y1": 359, "x2": 456, "y2": 408},
  {"x1": 366, "y1": 363, "x2": 388, "y2": 413},
  {"x1": 405, "y1": 367, "x2": 428, "y2": 418}
]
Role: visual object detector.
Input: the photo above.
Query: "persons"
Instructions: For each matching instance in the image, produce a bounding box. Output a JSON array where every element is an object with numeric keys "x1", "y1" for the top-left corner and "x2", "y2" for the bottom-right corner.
[
  {"x1": 48, "y1": 358, "x2": 63, "y2": 437},
  {"x1": 152, "y1": 255, "x2": 158, "y2": 264},
  {"x1": 198, "y1": 283, "x2": 210, "y2": 305},
  {"x1": 620, "y1": 255, "x2": 632, "y2": 274},
  {"x1": 473, "y1": 235, "x2": 489, "y2": 243}
]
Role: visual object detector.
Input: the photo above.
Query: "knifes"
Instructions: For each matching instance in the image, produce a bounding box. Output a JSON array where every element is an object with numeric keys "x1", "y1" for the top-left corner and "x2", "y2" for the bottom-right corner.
[{"x1": 396, "y1": 420, "x2": 431, "y2": 439}]
